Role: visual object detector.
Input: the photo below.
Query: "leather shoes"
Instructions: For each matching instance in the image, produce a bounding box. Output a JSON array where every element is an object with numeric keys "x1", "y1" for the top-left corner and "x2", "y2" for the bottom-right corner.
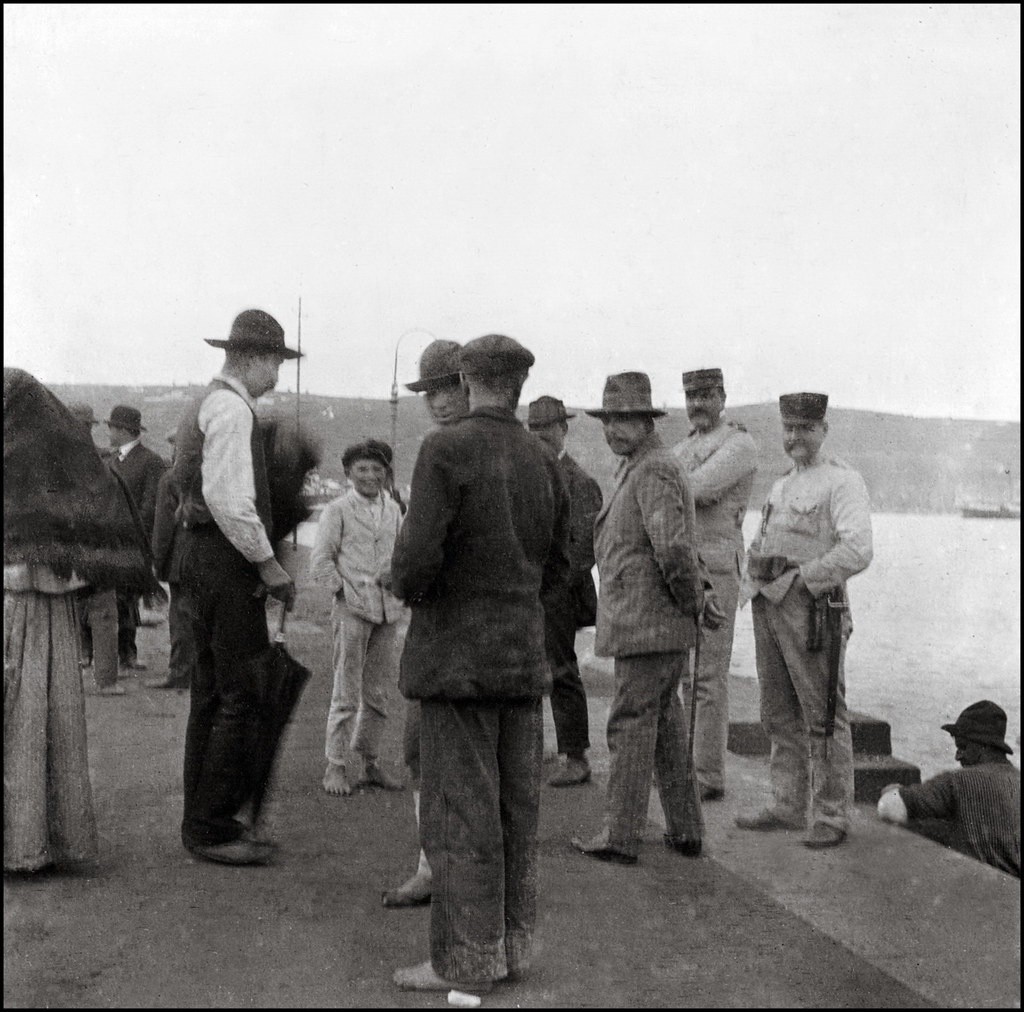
[
  {"x1": 664, "y1": 832, "x2": 702, "y2": 855},
  {"x1": 570, "y1": 835, "x2": 638, "y2": 864}
]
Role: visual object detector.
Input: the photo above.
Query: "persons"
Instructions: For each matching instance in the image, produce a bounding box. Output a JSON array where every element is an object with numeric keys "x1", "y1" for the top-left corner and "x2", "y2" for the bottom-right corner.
[
  {"x1": 2, "y1": 364, "x2": 162, "y2": 877},
  {"x1": 650, "y1": 371, "x2": 757, "y2": 804},
  {"x1": 876, "y1": 702, "x2": 1024, "y2": 875},
  {"x1": 372, "y1": 341, "x2": 563, "y2": 988},
  {"x1": 731, "y1": 391, "x2": 873, "y2": 848},
  {"x1": 527, "y1": 392, "x2": 600, "y2": 787},
  {"x1": 171, "y1": 311, "x2": 297, "y2": 862},
  {"x1": 315, "y1": 439, "x2": 410, "y2": 793},
  {"x1": 569, "y1": 371, "x2": 727, "y2": 866}
]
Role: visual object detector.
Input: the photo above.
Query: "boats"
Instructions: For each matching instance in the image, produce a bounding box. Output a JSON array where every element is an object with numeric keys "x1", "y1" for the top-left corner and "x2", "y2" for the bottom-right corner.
[{"x1": 963, "y1": 503, "x2": 1016, "y2": 518}]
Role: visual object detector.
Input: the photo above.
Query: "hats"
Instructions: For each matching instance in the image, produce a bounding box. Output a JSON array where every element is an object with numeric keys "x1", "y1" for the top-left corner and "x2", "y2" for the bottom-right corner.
[
  {"x1": 527, "y1": 396, "x2": 574, "y2": 425},
  {"x1": 682, "y1": 368, "x2": 723, "y2": 392},
  {"x1": 104, "y1": 406, "x2": 148, "y2": 433},
  {"x1": 451, "y1": 335, "x2": 535, "y2": 374},
  {"x1": 404, "y1": 340, "x2": 464, "y2": 391},
  {"x1": 779, "y1": 393, "x2": 829, "y2": 425},
  {"x1": 204, "y1": 309, "x2": 304, "y2": 359},
  {"x1": 940, "y1": 700, "x2": 1013, "y2": 754},
  {"x1": 585, "y1": 371, "x2": 666, "y2": 418},
  {"x1": 68, "y1": 400, "x2": 99, "y2": 424}
]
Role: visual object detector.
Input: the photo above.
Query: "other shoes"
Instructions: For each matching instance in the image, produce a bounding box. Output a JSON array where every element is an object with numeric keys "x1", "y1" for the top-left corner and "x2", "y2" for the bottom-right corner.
[
  {"x1": 120, "y1": 658, "x2": 146, "y2": 671},
  {"x1": 548, "y1": 760, "x2": 591, "y2": 786},
  {"x1": 183, "y1": 835, "x2": 278, "y2": 865},
  {"x1": 697, "y1": 780, "x2": 725, "y2": 801},
  {"x1": 145, "y1": 676, "x2": 189, "y2": 689},
  {"x1": 380, "y1": 877, "x2": 433, "y2": 905},
  {"x1": 734, "y1": 808, "x2": 806, "y2": 831},
  {"x1": 804, "y1": 820, "x2": 847, "y2": 847},
  {"x1": 81, "y1": 656, "x2": 90, "y2": 665}
]
